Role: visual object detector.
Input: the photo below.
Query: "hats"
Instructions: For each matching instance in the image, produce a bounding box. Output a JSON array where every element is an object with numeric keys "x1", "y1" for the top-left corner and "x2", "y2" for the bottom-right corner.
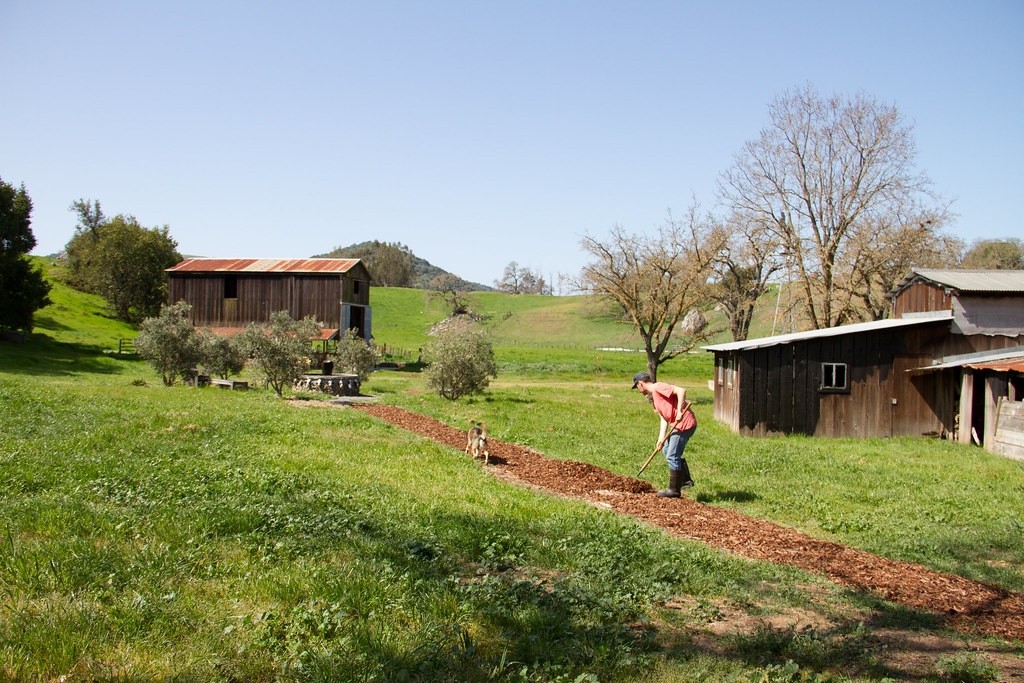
[{"x1": 631, "y1": 372, "x2": 650, "y2": 390}]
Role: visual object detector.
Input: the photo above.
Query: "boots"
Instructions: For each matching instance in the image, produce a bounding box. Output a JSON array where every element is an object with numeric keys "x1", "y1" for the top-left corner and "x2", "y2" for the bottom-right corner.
[
  {"x1": 681, "y1": 457, "x2": 694, "y2": 488},
  {"x1": 657, "y1": 469, "x2": 682, "y2": 498}
]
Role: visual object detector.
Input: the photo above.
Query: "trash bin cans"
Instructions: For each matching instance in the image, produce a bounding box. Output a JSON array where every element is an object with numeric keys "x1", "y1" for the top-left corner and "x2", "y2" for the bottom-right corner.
[{"x1": 321, "y1": 362, "x2": 333, "y2": 375}]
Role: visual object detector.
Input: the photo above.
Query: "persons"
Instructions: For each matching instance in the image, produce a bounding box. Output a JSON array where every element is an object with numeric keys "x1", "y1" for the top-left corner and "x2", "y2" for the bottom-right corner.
[{"x1": 630, "y1": 373, "x2": 698, "y2": 498}]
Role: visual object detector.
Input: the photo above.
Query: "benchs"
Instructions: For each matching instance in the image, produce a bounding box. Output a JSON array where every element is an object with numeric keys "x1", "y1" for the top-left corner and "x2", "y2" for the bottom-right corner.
[
  {"x1": 182, "y1": 370, "x2": 209, "y2": 388},
  {"x1": 211, "y1": 379, "x2": 248, "y2": 390}
]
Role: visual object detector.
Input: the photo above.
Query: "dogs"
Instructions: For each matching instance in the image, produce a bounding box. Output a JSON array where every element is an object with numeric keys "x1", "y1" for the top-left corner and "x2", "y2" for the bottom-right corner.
[{"x1": 465, "y1": 423, "x2": 489, "y2": 465}]
